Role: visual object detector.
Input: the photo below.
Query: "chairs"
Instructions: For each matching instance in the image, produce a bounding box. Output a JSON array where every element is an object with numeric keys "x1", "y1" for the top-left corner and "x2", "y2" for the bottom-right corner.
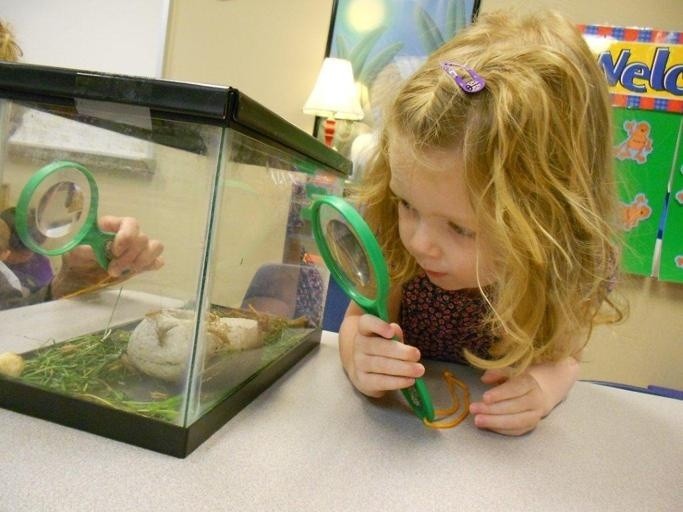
[{"x1": 239, "y1": 262, "x2": 351, "y2": 334}]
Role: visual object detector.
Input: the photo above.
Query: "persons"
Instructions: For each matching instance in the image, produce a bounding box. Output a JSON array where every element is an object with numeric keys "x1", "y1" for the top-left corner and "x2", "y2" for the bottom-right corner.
[
  {"x1": 0, "y1": 22, "x2": 162, "y2": 309},
  {"x1": 339, "y1": 6, "x2": 630, "y2": 437}
]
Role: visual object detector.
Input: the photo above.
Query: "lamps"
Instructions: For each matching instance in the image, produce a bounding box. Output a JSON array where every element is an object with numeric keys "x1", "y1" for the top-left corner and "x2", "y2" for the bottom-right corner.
[{"x1": 303, "y1": 57, "x2": 364, "y2": 147}]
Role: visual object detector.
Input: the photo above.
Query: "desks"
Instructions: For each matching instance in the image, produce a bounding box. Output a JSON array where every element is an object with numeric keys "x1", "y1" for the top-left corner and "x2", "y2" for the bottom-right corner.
[{"x1": 0, "y1": 286, "x2": 683, "y2": 512}]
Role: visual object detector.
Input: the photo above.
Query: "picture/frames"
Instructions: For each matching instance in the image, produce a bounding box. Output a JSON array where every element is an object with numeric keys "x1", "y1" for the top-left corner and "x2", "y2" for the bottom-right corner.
[{"x1": 313, "y1": 0, "x2": 481, "y2": 159}]
